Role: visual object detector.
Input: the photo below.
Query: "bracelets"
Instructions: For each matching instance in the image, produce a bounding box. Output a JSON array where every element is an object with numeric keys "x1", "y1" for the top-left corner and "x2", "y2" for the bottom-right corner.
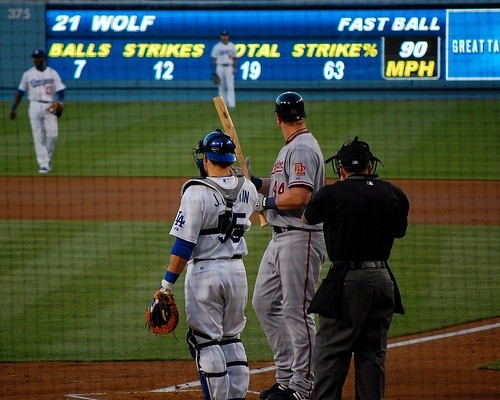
[
  {"x1": 11, "y1": 104, "x2": 17, "y2": 111},
  {"x1": 59, "y1": 99, "x2": 64, "y2": 102}
]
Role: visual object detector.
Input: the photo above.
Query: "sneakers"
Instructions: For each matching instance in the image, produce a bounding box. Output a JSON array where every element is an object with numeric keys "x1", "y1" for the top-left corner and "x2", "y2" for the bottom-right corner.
[
  {"x1": 259, "y1": 383, "x2": 288, "y2": 400},
  {"x1": 267, "y1": 388, "x2": 312, "y2": 400}
]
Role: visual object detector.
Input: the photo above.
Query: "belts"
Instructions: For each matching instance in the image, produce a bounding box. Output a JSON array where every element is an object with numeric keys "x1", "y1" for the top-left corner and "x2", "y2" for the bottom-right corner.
[
  {"x1": 273, "y1": 225, "x2": 322, "y2": 234},
  {"x1": 36, "y1": 99, "x2": 51, "y2": 104},
  {"x1": 331, "y1": 260, "x2": 386, "y2": 270},
  {"x1": 193, "y1": 254, "x2": 242, "y2": 262}
]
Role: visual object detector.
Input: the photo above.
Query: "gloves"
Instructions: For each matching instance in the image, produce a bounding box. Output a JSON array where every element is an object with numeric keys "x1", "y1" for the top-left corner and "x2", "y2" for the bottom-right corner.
[{"x1": 10, "y1": 112, "x2": 16, "y2": 119}]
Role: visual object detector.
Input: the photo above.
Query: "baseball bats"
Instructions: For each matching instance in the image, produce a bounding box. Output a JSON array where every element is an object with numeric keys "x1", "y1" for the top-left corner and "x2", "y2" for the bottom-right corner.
[{"x1": 212, "y1": 94, "x2": 270, "y2": 227}]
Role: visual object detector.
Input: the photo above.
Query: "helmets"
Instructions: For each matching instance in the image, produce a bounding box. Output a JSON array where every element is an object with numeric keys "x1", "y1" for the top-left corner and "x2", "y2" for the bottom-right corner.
[
  {"x1": 325, "y1": 136, "x2": 385, "y2": 175},
  {"x1": 275, "y1": 91, "x2": 307, "y2": 123},
  {"x1": 200, "y1": 131, "x2": 237, "y2": 162}
]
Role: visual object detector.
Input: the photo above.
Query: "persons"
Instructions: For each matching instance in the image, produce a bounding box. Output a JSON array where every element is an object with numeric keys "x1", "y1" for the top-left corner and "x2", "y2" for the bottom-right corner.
[
  {"x1": 210, "y1": 30, "x2": 237, "y2": 109},
  {"x1": 234, "y1": 92, "x2": 328, "y2": 400},
  {"x1": 10, "y1": 48, "x2": 66, "y2": 174},
  {"x1": 146, "y1": 130, "x2": 257, "y2": 400},
  {"x1": 302, "y1": 135, "x2": 410, "y2": 400}
]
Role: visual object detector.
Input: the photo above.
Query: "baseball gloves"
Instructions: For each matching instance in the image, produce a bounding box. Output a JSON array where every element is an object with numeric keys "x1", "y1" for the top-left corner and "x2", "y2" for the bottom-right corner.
[
  {"x1": 46, "y1": 101, "x2": 64, "y2": 117},
  {"x1": 144, "y1": 289, "x2": 179, "y2": 335}
]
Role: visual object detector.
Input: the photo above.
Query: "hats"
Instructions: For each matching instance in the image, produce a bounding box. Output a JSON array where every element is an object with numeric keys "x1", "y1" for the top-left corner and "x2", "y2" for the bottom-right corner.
[
  {"x1": 220, "y1": 30, "x2": 229, "y2": 36},
  {"x1": 30, "y1": 48, "x2": 45, "y2": 57}
]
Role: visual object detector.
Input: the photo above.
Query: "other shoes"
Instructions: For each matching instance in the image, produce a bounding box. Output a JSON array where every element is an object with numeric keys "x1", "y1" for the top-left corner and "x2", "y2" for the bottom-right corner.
[{"x1": 38, "y1": 166, "x2": 48, "y2": 174}]
